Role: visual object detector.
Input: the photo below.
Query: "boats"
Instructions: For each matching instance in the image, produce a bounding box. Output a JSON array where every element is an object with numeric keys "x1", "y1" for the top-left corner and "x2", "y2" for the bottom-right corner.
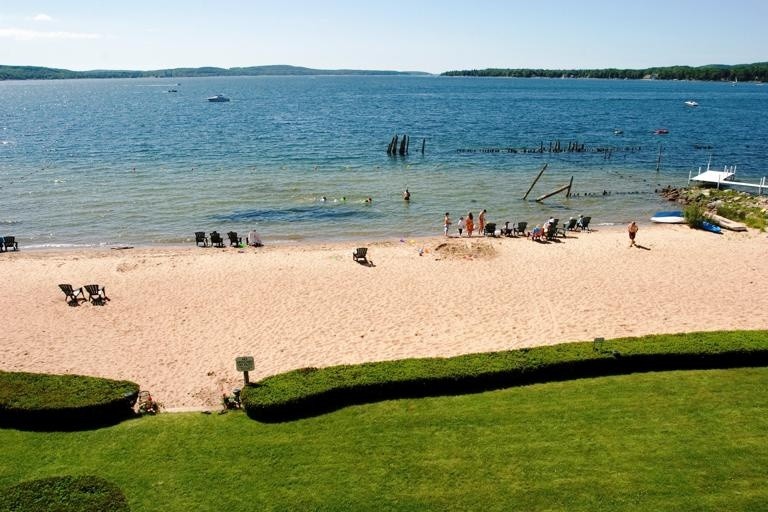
[
  {"x1": 207, "y1": 96, "x2": 229, "y2": 102},
  {"x1": 685, "y1": 101, "x2": 698, "y2": 106},
  {"x1": 651, "y1": 210, "x2": 746, "y2": 233}
]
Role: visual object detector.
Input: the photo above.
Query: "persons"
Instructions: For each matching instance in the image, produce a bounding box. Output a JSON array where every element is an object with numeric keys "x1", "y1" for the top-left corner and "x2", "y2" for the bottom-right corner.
[
  {"x1": 627, "y1": 221, "x2": 638, "y2": 247},
  {"x1": 444, "y1": 209, "x2": 585, "y2": 240},
  {"x1": 368, "y1": 197, "x2": 371, "y2": 202},
  {"x1": 402, "y1": 189, "x2": 410, "y2": 200}
]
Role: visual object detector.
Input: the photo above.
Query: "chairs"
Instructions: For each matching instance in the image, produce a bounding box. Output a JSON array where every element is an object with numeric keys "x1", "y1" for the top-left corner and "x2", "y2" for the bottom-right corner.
[
  {"x1": 501, "y1": 221, "x2": 527, "y2": 237},
  {"x1": 483, "y1": 223, "x2": 496, "y2": 235},
  {"x1": 4, "y1": 236, "x2": 18, "y2": 251},
  {"x1": 351, "y1": 247, "x2": 368, "y2": 261},
  {"x1": 545, "y1": 217, "x2": 590, "y2": 243},
  {"x1": 194, "y1": 230, "x2": 244, "y2": 248},
  {"x1": 58, "y1": 284, "x2": 110, "y2": 307}
]
